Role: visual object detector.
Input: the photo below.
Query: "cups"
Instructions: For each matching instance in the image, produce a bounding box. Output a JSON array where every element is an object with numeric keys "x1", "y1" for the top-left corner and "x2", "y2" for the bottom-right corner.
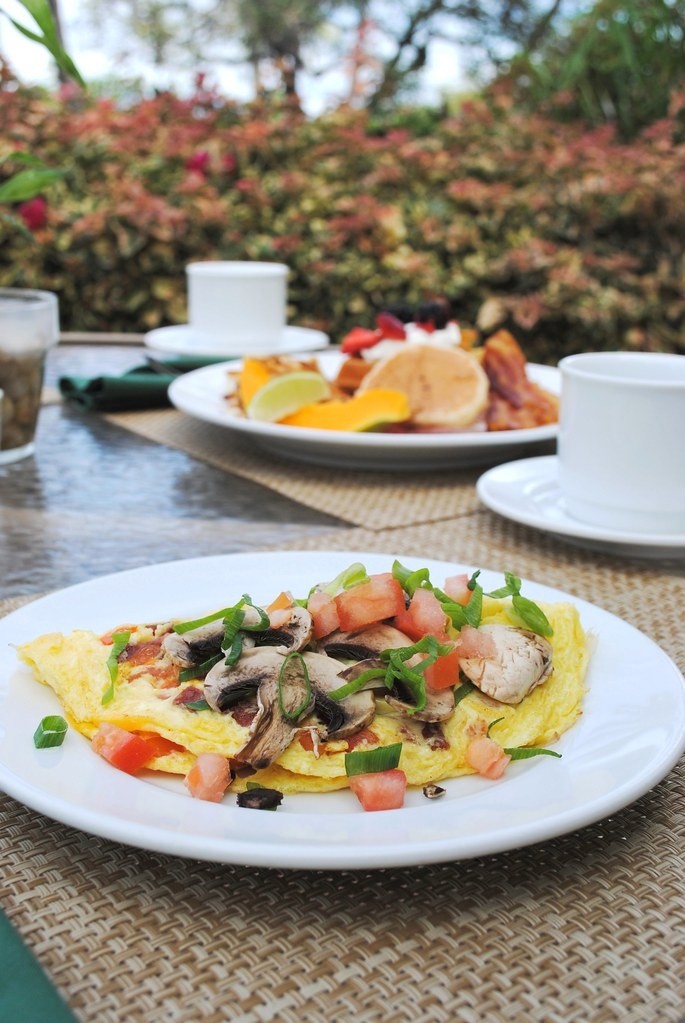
[
  {"x1": 0, "y1": 286, "x2": 59, "y2": 468},
  {"x1": 558, "y1": 351, "x2": 685, "y2": 535},
  {"x1": 185, "y1": 262, "x2": 288, "y2": 346}
]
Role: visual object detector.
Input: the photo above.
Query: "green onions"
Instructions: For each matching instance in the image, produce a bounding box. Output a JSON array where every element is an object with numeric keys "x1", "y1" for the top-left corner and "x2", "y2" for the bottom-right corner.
[{"x1": 34, "y1": 562, "x2": 562, "y2": 776}]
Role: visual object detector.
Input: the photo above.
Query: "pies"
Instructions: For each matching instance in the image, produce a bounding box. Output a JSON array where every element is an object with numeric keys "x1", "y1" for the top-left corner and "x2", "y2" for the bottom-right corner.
[{"x1": 16, "y1": 594, "x2": 587, "y2": 790}]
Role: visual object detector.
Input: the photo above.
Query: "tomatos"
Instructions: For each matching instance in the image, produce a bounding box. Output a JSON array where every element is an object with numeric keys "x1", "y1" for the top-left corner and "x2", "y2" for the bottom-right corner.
[{"x1": 90, "y1": 573, "x2": 512, "y2": 813}]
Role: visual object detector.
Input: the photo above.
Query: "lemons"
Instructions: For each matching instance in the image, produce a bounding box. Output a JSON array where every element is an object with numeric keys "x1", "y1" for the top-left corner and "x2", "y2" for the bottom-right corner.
[{"x1": 247, "y1": 369, "x2": 330, "y2": 422}]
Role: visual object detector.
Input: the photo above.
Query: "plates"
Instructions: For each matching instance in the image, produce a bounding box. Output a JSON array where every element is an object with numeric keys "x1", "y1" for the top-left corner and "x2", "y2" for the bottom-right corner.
[
  {"x1": 167, "y1": 351, "x2": 559, "y2": 470},
  {"x1": 144, "y1": 323, "x2": 329, "y2": 366},
  {"x1": 0, "y1": 551, "x2": 685, "y2": 869},
  {"x1": 476, "y1": 455, "x2": 685, "y2": 559}
]
